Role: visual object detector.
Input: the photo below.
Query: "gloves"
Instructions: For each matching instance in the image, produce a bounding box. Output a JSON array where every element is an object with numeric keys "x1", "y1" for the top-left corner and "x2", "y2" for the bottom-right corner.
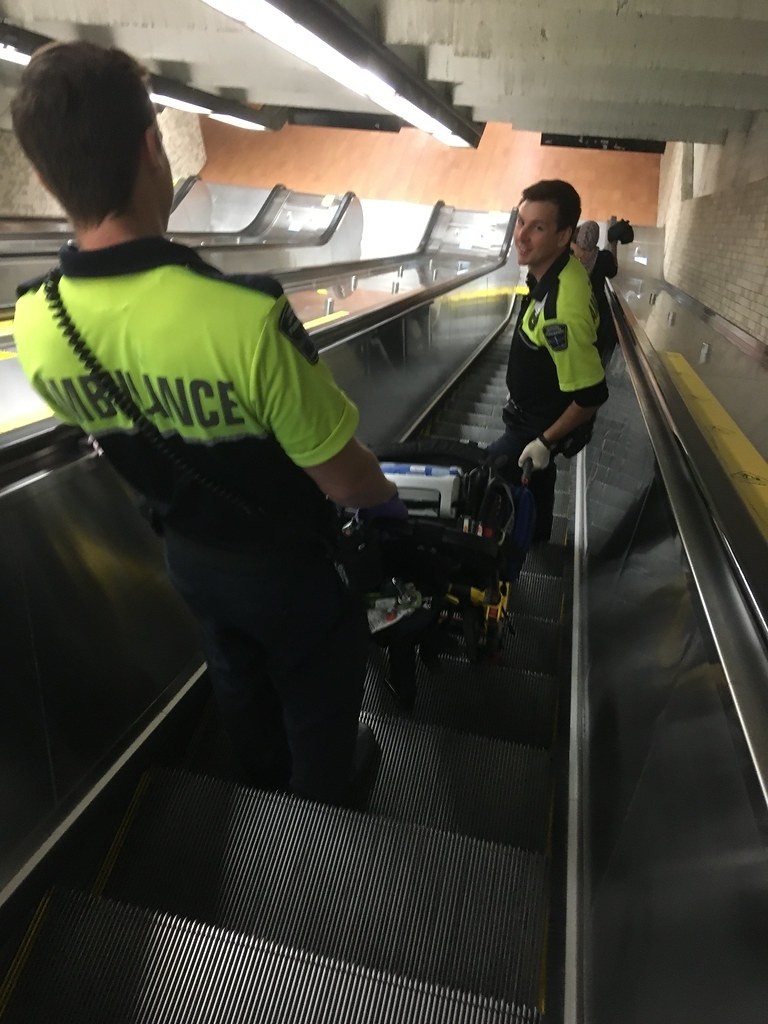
[
  {"x1": 518, "y1": 433, "x2": 551, "y2": 471},
  {"x1": 355, "y1": 491, "x2": 409, "y2": 524}
]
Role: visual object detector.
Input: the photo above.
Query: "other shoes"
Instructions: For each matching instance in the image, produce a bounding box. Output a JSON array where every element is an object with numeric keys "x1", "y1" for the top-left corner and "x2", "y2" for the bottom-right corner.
[{"x1": 289, "y1": 723, "x2": 380, "y2": 812}]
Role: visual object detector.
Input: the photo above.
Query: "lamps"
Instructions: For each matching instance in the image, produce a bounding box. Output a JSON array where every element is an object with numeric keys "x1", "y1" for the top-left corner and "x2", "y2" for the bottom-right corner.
[
  {"x1": 0, "y1": 22, "x2": 270, "y2": 133},
  {"x1": 203, "y1": 0, "x2": 480, "y2": 149},
  {"x1": 288, "y1": 107, "x2": 402, "y2": 134},
  {"x1": 261, "y1": 105, "x2": 288, "y2": 132},
  {"x1": 541, "y1": 133, "x2": 666, "y2": 155}
]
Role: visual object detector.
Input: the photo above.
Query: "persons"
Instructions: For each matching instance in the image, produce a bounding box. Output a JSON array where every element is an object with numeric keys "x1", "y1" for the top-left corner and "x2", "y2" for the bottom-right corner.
[
  {"x1": 11, "y1": 42, "x2": 398, "y2": 809},
  {"x1": 572, "y1": 220, "x2": 619, "y2": 368},
  {"x1": 485, "y1": 179, "x2": 609, "y2": 551}
]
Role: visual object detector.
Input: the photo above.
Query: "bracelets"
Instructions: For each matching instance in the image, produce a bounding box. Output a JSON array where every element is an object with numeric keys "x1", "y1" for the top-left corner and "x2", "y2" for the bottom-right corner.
[{"x1": 537, "y1": 432, "x2": 551, "y2": 451}]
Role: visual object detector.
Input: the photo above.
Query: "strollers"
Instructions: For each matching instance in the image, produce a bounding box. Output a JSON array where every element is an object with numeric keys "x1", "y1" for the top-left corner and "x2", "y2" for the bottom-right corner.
[{"x1": 336, "y1": 435, "x2": 540, "y2": 712}]
